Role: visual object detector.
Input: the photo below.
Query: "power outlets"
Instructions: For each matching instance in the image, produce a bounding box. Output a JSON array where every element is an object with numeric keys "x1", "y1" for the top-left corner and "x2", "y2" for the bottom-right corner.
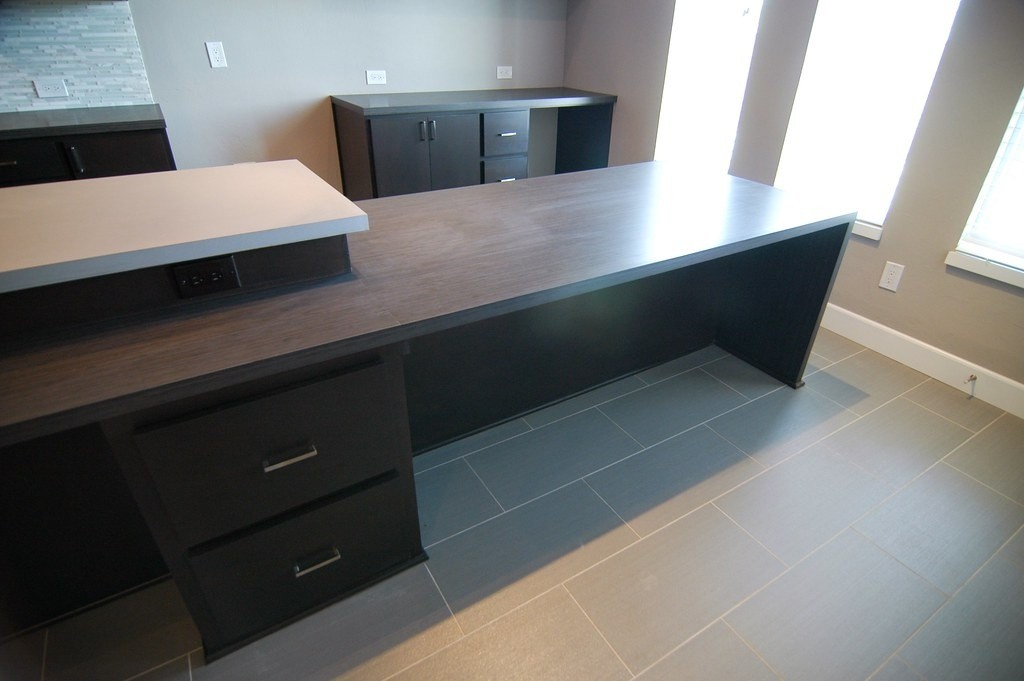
[
  {"x1": 879, "y1": 260, "x2": 905, "y2": 292},
  {"x1": 366, "y1": 69, "x2": 387, "y2": 83},
  {"x1": 206, "y1": 41, "x2": 227, "y2": 67},
  {"x1": 497, "y1": 65, "x2": 513, "y2": 79}
]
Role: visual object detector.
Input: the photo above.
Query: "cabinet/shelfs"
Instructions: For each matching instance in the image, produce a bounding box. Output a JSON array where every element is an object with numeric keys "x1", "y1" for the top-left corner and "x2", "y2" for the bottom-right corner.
[
  {"x1": 0, "y1": 103, "x2": 177, "y2": 188},
  {"x1": 1, "y1": 159, "x2": 857, "y2": 665},
  {"x1": 332, "y1": 103, "x2": 480, "y2": 201},
  {"x1": 480, "y1": 109, "x2": 529, "y2": 184}
]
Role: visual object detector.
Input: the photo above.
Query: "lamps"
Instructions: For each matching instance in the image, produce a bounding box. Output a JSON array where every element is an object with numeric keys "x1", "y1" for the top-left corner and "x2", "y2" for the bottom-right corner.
[{"x1": 34, "y1": 77, "x2": 70, "y2": 97}]
